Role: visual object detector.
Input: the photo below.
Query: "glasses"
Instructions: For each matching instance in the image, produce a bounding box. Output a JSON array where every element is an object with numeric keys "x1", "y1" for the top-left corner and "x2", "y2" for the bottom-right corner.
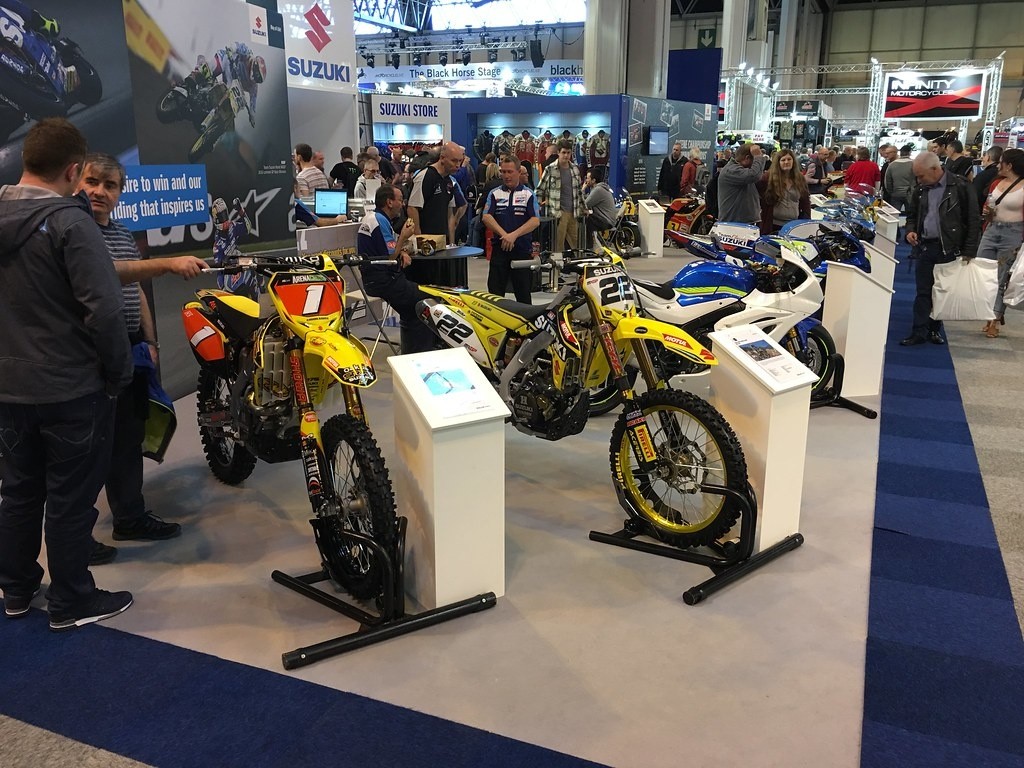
[
  {"x1": 402, "y1": 171, "x2": 409, "y2": 174},
  {"x1": 366, "y1": 168, "x2": 380, "y2": 173}
]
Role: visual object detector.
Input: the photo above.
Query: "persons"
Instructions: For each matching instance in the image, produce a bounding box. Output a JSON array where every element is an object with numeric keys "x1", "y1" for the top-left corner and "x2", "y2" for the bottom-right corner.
[
  {"x1": 464, "y1": 163, "x2": 503, "y2": 259},
  {"x1": 189, "y1": 42, "x2": 267, "y2": 125},
  {"x1": 972, "y1": 145, "x2": 1024, "y2": 337},
  {"x1": 475, "y1": 153, "x2": 497, "y2": 187},
  {"x1": 897, "y1": 153, "x2": 981, "y2": 346},
  {"x1": 289, "y1": 141, "x2": 331, "y2": 196},
  {"x1": 581, "y1": 168, "x2": 616, "y2": 249},
  {"x1": 657, "y1": 137, "x2": 973, "y2": 238},
  {"x1": 327, "y1": 146, "x2": 363, "y2": 198},
  {"x1": 0, "y1": 119, "x2": 134, "y2": 630},
  {"x1": 292, "y1": 177, "x2": 347, "y2": 226},
  {"x1": 483, "y1": 156, "x2": 541, "y2": 306},
  {"x1": 354, "y1": 147, "x2": 403, "y2": 199},
  {"x1": 357, "y1": 185, "x2": 443, "y2": 355},
  {"x1": 534, "y1": 142, "x2": 589, "y2": 253},
  {"x1": 393, "y1": 140, "x2": 470, "y2": 288},
  {"x1": 73, "y1": 150, "x2": 211, "y2": 567}
]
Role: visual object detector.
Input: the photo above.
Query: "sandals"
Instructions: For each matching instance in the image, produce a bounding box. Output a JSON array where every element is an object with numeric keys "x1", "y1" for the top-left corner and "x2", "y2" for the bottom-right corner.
[
  {"x1": 982, "y1": 320, "x2": 992, "y2": 333},
  {"x1": 985, "y1": 320, "x2": 1001, "y2": 338}
]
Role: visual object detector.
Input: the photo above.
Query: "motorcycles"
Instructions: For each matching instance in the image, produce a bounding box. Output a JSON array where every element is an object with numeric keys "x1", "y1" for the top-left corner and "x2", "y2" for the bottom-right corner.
[
  {"x1": 598, "y1": 169, "x2": 883, "y2": 397},
  {"x1": 410, "y1": 210, "x2": 749, "y2": 548},
  {"x1": 184, "y1": 245, "x2": 401, "y2": 601},
  {"x1": 158, "y1": 47, "x2": 255, "y2": 169},
  {"x1": 0, "y1": 20, "x2": 104, "y2": 140}
]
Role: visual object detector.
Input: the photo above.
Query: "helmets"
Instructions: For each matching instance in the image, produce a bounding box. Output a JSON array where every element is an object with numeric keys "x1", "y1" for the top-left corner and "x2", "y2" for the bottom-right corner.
[
  {"x1": 211, "y1": 197, "x2": 233, "y2": 231},
  {"x1": 247, "y1": 56, "x2": 267, "y2": 85}
]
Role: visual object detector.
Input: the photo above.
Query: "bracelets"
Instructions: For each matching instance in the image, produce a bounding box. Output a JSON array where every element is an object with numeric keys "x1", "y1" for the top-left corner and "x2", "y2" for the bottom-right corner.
[{"x1": 145, "y1": 340, "x2": 160, "y2": 350}]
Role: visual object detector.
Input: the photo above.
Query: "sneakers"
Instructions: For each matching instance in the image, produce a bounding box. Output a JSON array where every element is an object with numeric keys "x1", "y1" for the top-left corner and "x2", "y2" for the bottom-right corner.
[
  {"x1": 85, "y1": 537, "x2": 118, "y2": 567},
  {"x1": 2, "y1": 584, "x2": 44, "y2": 618},
  {"x1": 113, "y1": 508, "x2": 182, "y2": 542},
  {"x1": 49, "y1": 588, "x2": 133, "y2": 632}
]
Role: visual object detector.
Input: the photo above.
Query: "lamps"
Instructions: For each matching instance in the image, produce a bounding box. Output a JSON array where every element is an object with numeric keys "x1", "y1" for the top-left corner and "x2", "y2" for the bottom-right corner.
[
  {"x1": 739, "y1": 63, "x2": 780, "y2": 90},
  {"x1": 359, "y1": 27, "x2": 545, "y2": 69}
]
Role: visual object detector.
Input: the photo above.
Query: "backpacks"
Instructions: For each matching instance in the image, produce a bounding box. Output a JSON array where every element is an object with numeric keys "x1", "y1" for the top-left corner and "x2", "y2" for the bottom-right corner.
[{"x1": 689, "y1": 160, "x2": 711, "y2": 188}]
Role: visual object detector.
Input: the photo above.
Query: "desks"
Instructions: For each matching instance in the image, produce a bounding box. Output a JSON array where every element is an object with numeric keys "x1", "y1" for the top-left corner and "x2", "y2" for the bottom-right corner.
[{"x1": 406, "y1": 245, "x2": 484, "y2": 290}]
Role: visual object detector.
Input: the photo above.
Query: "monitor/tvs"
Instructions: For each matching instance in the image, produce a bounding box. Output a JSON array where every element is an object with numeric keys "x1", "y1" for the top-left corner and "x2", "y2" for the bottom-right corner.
[
  {"x1": 314, "y1": 188, "x2": 349, "y2": 218},
  {"x1": 648, "y1": 126, "x2": 669, "y2": 155}
]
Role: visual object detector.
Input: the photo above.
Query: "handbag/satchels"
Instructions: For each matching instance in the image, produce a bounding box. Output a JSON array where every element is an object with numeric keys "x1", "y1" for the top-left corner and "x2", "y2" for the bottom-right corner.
[
  {"x1": 930, "y1": 255, "x2": 1000, "y2": 322},
  {"x1": 1003, "y1": 242, "x2": 1024, "y2": 309}
]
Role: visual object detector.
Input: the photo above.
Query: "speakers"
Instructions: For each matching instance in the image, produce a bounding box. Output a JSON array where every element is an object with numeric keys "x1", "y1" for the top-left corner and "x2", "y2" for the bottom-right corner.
[{"x1": 530, "y1": 39, "x2": 545, "y2": 69}]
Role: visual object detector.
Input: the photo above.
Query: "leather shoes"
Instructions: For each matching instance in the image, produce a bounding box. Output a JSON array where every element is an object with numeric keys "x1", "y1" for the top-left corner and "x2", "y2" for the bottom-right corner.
[
  {"x1": 929, "y1": 331, "x2": 945, "y2": 344},
  {"x1": 900, "y1": 332, "x2": 927, "y2": 346}
]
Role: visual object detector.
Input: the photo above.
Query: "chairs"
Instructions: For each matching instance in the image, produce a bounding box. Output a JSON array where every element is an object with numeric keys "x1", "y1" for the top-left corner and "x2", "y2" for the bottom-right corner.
[
  {"x1": 594, "y1": 204, "x2": 628, "y2": 258},
  {"x1": 333, "y1": 252, "x2": 401, "y2": 360}
]
